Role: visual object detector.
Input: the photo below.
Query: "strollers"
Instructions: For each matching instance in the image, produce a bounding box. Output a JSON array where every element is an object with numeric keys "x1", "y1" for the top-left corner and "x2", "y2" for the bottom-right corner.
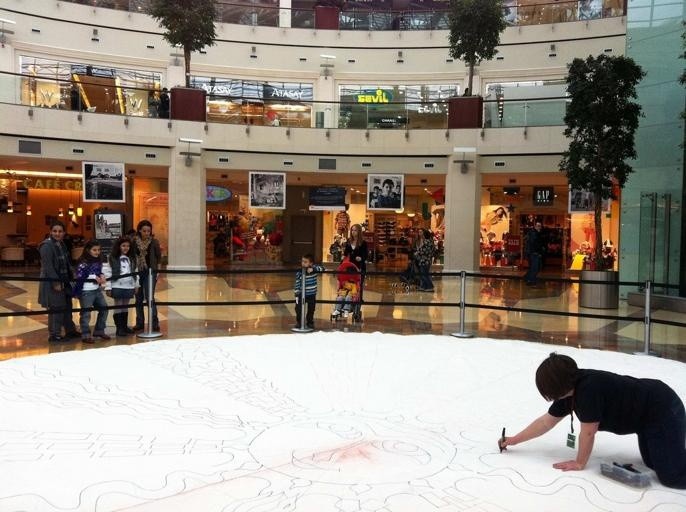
[{"x1": 330, "y1": 259, "x2": 362, "y2": 324}]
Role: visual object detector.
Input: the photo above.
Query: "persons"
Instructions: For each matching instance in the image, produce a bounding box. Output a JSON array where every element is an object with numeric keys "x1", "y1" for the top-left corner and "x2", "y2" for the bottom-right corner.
[
  {"x1": 158, "y1": 88, "x2": 170, "y2": 118},
  {"x1": 371, "y1": 178, "x2": 401, "y2": 208},
  {"x1": 36, "y1": 217, "x2": 161, "y2": 345},
  {"x1": 483, "y1": 207, "x2": 507, "y2": 229},
  {"x1": 479, "y1": 229, "x2": 512, "y2": 267},
  {"x1": 213, "y1": 211, "x2": 251, "y2": 256},
  {"x1": 390, "y1": 13, "x2": 405, "y2": 30},
  {"x1": 344, "y1": 224, "x2": 368, "y2": 315},
  {"x1": 332, "y1": 279, "x2": 358, "y2": 318},
  {"x1": 295, "y1": 254, "x2": 325, "y2": 329},
  {"x1": 462, "y1": 87, "x2": 469, "y2": 98},
  {"x1": 582, "y1": 214, "x2": 596, "y2": 246},
  {"x1": 335, "y1": 204, "x2": 350, "y2": 238},
  {"x1": 329, "y1": 235, "x2": 344, "y2": 263},
  {"x1": 270, "y1": 114, "x2": 282, "y2": 128},
  {"x1": 572, "y1": 187, "x2": 593, "y2": 209},
  {"x1": 147, "y1": 89, "x2": 159, "y2": 118},
  {"x1": 498, "y1": 352, "x2": 685, "y2": 491},
  {"x1": 417, "y1": 230, "x2": 435, "y2": 292},
  {"x1": 409, "y1": 228, "x2": 423, "y2": 286},
  {"x1": 524, "y1": 220, "x2": 543, "y2": 284}
]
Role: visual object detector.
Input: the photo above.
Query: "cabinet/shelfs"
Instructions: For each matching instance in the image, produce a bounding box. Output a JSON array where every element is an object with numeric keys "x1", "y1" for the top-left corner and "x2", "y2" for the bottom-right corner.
[
  {"x1": 366, "y1": 214, "x2": 413, "y2": 266},
  {"x1": 513, "y1": 208, "x2": 566, "y2": 272}
]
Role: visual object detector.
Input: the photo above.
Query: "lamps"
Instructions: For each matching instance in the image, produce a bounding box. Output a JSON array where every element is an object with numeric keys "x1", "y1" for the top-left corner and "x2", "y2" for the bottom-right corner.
[
  {"x1": 453, "y1": 144, "x2": 477, "y2": 173},
  {"x1": 177, "y1": 136, "x2": 203, "y2": 167},
  {"x1": 6, "y1": 176, "x2": 13, "y2": 213},
  {"x1": 58, "y1": 190, "x2": 63, "y2": 217},
  {"x1": 76, "y1": 191, "x2": 82, "y2": 216},
  {"x1": 25, "y1": 189, "x2": 32, "y2": 216},
  {"x1": 67, "y1": 191, "x2": 73, "y2": 215}
]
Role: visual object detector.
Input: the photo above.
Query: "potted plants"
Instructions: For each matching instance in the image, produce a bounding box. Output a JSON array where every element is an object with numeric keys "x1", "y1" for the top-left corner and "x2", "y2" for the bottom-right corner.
[
  {"x1": 437, "y1": 1, "x2": 511, "y2": 129},
  {"x1": 559, "y1": 54, "x2": 649, "y2": 309},
  {"x1": 143, "y1": 0, "x2": 219, "y2": 122}
]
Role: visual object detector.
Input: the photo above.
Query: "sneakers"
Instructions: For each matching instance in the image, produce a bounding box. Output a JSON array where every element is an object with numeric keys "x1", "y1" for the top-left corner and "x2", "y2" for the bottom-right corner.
[
  {"x1": 332, "y1": 310, "x2": 340, "y2": 315},
  {"x1": 82, "y1": 337, "x2": 95, "y2": 343},
  {"x1": 418, "y1": 287, "x2": 423, "y2": 290},
  {"x1": 425, "y1": 289, "x2": 434, "y2": 292},
  {"x1": 295, "y1": 323, "x2": 301, "y2": 327},
  {"x1": 48, "y1": 335, "x2": 69, "y2": 341},
  {"x1": 65, "y1": 331, "x2": 81, "y2": 337},
  {"x1": 94, "y1": 334, "x2": 110, "y2": 340},
  {"x1": 307, "y1": 322, "x2": 315, "y2": 328},
  {"x1": 344, "y1": 312, "x2": 348, "y2": 318}
]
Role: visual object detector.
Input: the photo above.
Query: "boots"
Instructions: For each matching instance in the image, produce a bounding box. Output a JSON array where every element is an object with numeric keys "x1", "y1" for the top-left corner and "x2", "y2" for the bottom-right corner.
[
  {"x1": 122, "y1": 313, "x2": 134, "y2": 333},
  {"x1": 153, "y1": 317, "x2": 158, "y2": 331},
  {"x1": 113, "y1": 312, "x2": 127, "y2": 336},
  {"x1": 133, "y1": 317, "x2": 144, "y2": 331}
]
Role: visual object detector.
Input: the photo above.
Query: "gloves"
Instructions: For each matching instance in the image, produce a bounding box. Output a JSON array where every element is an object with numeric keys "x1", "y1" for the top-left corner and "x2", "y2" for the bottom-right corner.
[{"x1": 296, "y1": 297, "x2": 299, "y2": 304}]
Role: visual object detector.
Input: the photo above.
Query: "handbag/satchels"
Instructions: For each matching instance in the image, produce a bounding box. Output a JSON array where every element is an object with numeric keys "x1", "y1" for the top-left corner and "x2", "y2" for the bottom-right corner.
[{"x1": 66, "y1": 281, "x2": 77, "y2": 297}]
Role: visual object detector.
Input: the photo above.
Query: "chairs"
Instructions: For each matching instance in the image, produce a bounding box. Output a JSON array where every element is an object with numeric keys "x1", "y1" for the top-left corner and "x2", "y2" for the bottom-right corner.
[
  {"x1": 70, "y1": 246, "x2": 84, "y2": 266},
  {"x1": 0, "y1": 246, "x2": 27, "y2": 269}
]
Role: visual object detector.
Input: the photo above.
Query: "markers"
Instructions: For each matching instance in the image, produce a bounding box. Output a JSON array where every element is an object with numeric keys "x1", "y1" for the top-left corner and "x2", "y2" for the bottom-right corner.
[
  {"x1": 612, "y1": 462, "x2": 642, "y2": 473},
  {"x1": 500, "y1": 428, "x2": 506, "y2": 453}
]
